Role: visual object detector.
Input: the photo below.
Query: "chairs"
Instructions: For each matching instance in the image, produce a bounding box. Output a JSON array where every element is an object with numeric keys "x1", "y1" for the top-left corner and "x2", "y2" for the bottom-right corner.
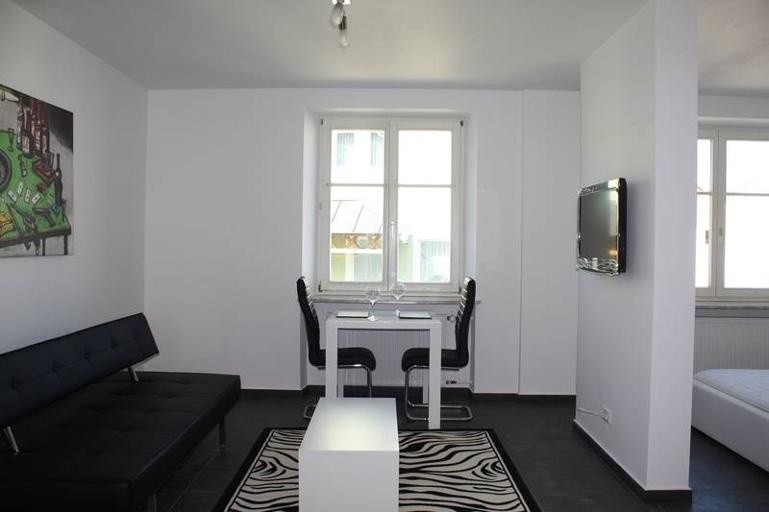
[{"x1": 293, "y1": 272, "x2": 478, "y2": 420}]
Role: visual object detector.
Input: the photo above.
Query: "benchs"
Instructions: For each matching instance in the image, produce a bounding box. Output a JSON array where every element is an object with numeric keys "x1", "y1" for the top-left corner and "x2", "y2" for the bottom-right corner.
[{"x1": 3, "y1": 312, "x2": 240, "y2": 509}]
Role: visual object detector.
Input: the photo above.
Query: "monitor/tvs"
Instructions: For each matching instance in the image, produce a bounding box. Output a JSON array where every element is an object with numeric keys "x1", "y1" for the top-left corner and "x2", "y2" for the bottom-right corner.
[{"x1": 576, "y1": 177, "x2": 627, "y2": 276}]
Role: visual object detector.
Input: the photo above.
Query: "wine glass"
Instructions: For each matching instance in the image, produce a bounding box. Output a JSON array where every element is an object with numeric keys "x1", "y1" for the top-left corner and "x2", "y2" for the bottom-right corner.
[{"x1": 367, "y1": 287, "x2": 380, "y2": 322}]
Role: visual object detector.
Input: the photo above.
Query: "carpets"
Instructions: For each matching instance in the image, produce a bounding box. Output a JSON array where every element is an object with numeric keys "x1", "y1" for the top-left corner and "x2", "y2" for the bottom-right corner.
[{"x1": 218, "y1": 425, "x2": 539, "y2": 512}]
